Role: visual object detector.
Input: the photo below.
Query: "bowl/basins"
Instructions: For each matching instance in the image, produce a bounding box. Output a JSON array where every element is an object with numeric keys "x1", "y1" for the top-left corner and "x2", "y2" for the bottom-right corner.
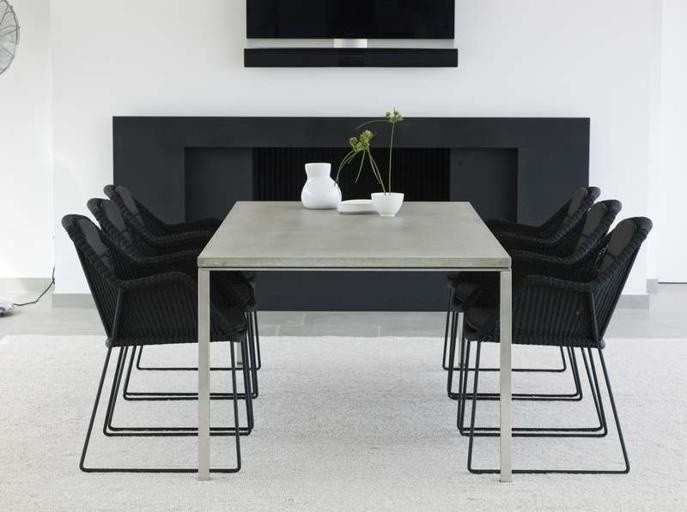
[{"x1": 370, "y1": 192, "x2": 405, "y2": 217}]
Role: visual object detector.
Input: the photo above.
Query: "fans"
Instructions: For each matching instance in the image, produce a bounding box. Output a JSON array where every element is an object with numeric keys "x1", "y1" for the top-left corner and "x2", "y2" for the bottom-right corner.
[{"x1": 0, "y1": 0, "x2": 56, "y2": 321}]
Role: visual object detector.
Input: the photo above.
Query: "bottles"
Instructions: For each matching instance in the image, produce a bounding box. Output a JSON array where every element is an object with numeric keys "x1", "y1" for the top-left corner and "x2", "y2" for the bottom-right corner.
[{"x1": 300, "y1": 161, "x2": 342, "y2": 208}]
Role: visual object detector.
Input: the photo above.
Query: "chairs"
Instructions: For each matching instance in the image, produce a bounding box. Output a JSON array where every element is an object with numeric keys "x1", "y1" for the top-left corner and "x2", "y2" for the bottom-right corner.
[
  {"x1": 446, "y1": 198, "x2": 624, "y2": 441},
  {"x1": 440, "y1": 185, "x2": 602, "y2": 399},
  {"x1": 102, "y1": 183, "x2": 262, "y2": 397},
  {"x1": 86, "y1": 196, "x2": 258, "y2": 431},
  {"x1": 458, "y1": 216, "x2": 654, "y2": 477},
  {"x1": 60, "y1": 213, "x2": 253, "y2": 474}
]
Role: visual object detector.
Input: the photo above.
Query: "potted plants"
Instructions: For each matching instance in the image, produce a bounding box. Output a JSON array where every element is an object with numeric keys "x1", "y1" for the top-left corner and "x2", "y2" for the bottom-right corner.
[{"x1": 333, "y1": 107, "x2": 405, "y2": 217}]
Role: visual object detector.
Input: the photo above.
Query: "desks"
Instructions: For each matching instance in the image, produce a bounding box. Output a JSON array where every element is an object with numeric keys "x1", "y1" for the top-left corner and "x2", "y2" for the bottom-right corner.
[{"x1": 191, "y1": 194, "x2": 515, "y2": 484}]
